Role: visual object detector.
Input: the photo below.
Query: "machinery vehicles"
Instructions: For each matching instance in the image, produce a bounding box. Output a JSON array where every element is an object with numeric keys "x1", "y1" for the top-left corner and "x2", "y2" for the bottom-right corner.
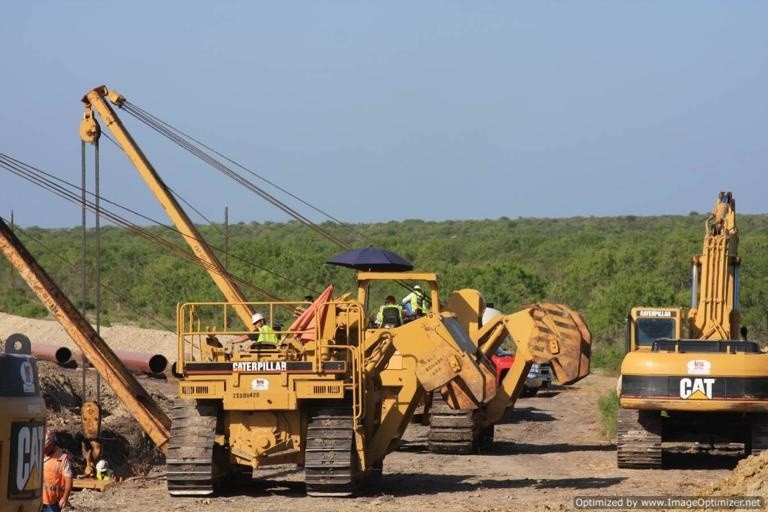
[
  {"x1": 0, "y1": 353, "x2": 48, "y2": 510},
  {"x1": 0, "y1": 150, "x2": 498, "y2": 500},
  {"x1": 616, "y1": 191, "x2": 768, "y2": 469},
  {"x1": 78, "y1": 83, "x2": 593, "y2": 456}
]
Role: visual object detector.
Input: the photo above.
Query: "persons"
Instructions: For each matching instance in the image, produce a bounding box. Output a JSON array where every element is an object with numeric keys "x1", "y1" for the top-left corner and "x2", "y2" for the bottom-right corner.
[
  {"x1": 41, "y1": 428, "x2": 73, "y2": 512},
  {"x1": 401, "y1": 285, "x2": 429, "y2": 317},
  {"x1": 375, "y1": 295, "x2": 404, "y2": 328},
  {"x1": 226, "y1": 313, "x2": 279, "y2": 347},
  {"x1": 95, "y1": 460, "x2": 118, "y2": 483}
]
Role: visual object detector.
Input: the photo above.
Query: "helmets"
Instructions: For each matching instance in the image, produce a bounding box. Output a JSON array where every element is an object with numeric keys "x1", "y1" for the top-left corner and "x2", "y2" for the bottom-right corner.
[
  {"x1": 252, "y1": 313, "x2": 263, "y2": 324},
  {"x1": 44, "y1": 432, "x2": 57, "y2": 448}
]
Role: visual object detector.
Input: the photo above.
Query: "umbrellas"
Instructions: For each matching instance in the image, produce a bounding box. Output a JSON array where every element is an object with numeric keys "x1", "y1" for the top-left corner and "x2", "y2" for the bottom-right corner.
[{"x1": 326, "y1": 246, "x2": 413, "y2": 312}]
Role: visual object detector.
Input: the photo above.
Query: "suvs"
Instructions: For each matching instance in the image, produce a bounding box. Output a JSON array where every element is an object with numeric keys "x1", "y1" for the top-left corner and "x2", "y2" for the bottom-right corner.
[{"x1": 485, "y1": 341, "x2": 553, "y2": 397}]
